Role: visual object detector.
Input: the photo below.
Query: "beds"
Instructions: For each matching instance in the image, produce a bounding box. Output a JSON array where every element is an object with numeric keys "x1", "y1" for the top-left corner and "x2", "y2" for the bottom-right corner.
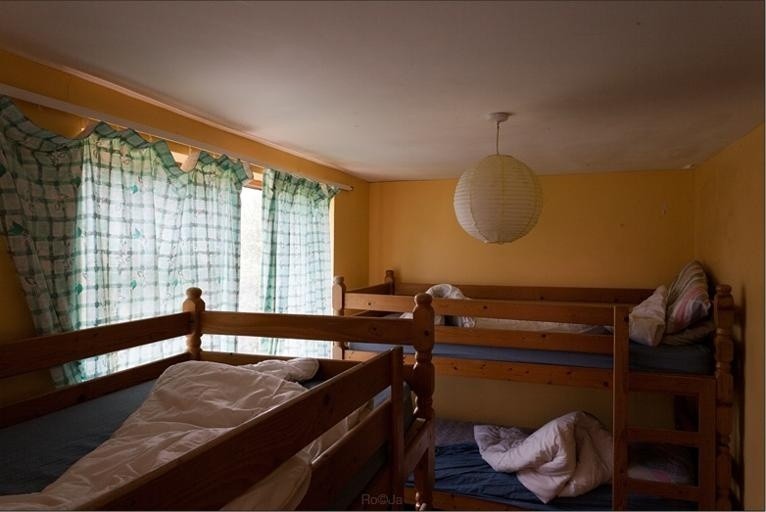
[
  {"x1": 332, "y1": 270, "x2": 737, "y2": 512},
  {"x1": 0, "y1": 287, "x2": 438, "y2": 511}
]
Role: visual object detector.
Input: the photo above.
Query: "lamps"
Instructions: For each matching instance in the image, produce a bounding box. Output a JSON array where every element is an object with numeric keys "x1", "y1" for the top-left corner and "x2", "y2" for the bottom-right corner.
[{"x1": 453, "y1": 114, "x2": 545, "y2": 245}]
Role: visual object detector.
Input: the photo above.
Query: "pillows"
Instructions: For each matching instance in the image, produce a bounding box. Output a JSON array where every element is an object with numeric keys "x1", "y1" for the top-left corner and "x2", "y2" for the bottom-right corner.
[
  {"x1": 665, "y1": 258, "x2": 711, "y2": 334},
  {"x1": 626, "y1": 442, "x2": 696, "y2": 486},
  {"x1": 663, "y1": 313, "x2": 715, "y2": 346}
]
